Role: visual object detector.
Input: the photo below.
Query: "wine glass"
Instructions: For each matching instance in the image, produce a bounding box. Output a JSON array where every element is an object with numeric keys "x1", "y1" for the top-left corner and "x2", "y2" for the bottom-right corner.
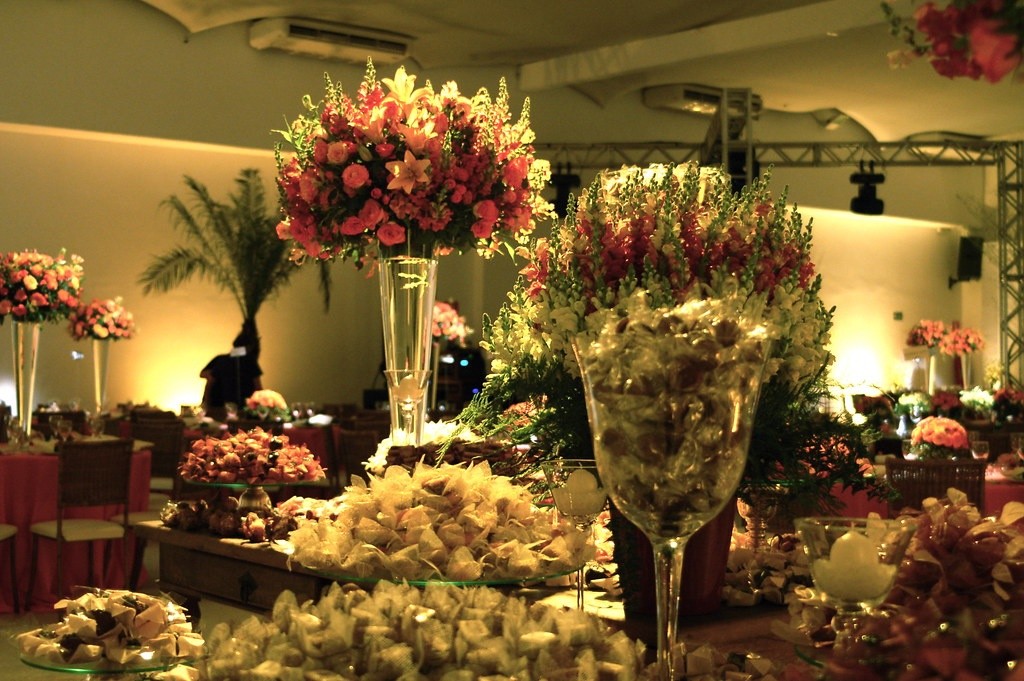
[
  {"x1": 792, "y1": 515, "x2": 919, "y2": 677},
  {"x1": 540, "y1": 458, "x2": 608, "y2": 612},
  {"x1": 224, "y1": 400, "x2": 319, "y2": 424},
  {"x1": 385, "y1": 370, "x2": 433, "y2": 447},
  {"x1": 4, "y1": 414, "x2": 105, "y2": 454}
]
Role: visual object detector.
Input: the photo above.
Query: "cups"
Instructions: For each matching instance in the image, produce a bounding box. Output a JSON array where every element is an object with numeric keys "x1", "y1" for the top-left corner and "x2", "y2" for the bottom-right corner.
[
  {"x1": 901, "y1": 429, "x2": 1024, "y2": 479},
  {"x1": 375, "y1": 401, "x2": 469, "y2": 412}
]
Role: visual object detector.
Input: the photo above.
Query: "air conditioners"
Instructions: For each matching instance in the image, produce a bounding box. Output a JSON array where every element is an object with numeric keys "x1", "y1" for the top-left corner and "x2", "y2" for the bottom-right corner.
[
  {"x1": 645, "y1": 82, "x2": 763, "y2": 121},
  {"x1": 249, "y1": 14, "x2": 416, "y2": 68}
]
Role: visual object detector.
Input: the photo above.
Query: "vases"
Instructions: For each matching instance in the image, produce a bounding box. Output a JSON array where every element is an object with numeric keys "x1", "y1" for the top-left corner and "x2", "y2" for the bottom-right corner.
[
  {"x1": 374, "y1": 253, "x2": 438, "y2": 448},
  {"x1": 10, "y1": 319, "x2": 42, "y2": 441},
  {"x1": 925, "y1": 346, "x2": 939, "y2": 399},
  {"x1": 960, "y1": 353, "x2": 974, "y2": 391},
  {"x1": 92, "y1": 336, "x2": 112, "y2": 406}
]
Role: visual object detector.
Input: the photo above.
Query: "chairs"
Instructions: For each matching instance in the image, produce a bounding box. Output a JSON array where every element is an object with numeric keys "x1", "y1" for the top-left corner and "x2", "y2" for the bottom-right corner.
[
  {"x1": 325, "y1": 404, "x2": 358, "y2": 429},
  {"x1": 884, "y1": 457, "x2": 987, "y2": 517},
  {"x1": 339, "y1": 428, "x2": 380, "y2": 482},
  {"x1": 131, "y1": 410, "x2": 177, "y2": 424},
  {"x1": 133, "y1": 424, "x2": 186, "y2": 501},
  {"x1": 0, "y1": 523, "x2": 19, "y2": 616},
  {"x1": 24, "y1": 438, "x2": 134, "y2": 624},
  {"x1": 227, "y1": 419, "x2": 285, "y2": 450},
  {"x1": 32, "y1": 411, "x2": 86, "y2": 453},
  {"x1": 366, "y1": 410, "x2": 391, "y2": 440}
]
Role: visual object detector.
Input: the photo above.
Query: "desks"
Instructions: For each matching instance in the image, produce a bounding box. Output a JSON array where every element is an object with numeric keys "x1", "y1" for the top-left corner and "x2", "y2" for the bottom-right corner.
[
  {"x1": 133, "y1": 516, "x2": 377, "y2": 626},
  {"x1": 85, "y1": 418, "x2": 132, "y2": 439},
  {"x1": 0, "y1": 431, "x2": 153, "y2": 621},
  {"x1": 182, "y1": 424, "x2": 345, "y2": 480},
  {"x1": 767, "y1": 474, "x2": 1024, "y2": 544}
]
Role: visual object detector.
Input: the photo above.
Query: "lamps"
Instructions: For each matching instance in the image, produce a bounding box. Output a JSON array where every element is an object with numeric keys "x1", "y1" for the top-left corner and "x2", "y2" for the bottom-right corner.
[
  {"x1": 948, "y1": 235, "x2": 985, "y2": 292},
  {"x1": 848, "y1": 162, "x2": 887, "y2": 215},
  {"x1": 545, "y1": 173, "x2": 581, "y2": 220}
]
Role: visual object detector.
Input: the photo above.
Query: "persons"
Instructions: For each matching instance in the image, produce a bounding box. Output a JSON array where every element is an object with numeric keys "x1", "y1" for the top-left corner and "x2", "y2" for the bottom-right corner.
[
  {"x1": 200, "y1": 329, "x2": 263, "y2": 427},
  {"x1": 871, "y1": 393, "x2": 903, "y2": 459},
  {"x1": 455, "y1": 349, "x2": 486, "y2": 411}
]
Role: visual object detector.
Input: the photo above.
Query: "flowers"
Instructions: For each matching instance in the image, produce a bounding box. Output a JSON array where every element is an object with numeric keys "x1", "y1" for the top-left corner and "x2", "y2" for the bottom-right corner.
[
  {"x1": 993, "y1": 383, "x2": 1024, "y2": 420},
  {"x1": 900, "y1": 392, "x2": 934, "y2": 416},
  {"x1": 878, "y1": 0, "x2": 1024, "y2": 84},
  {"x1": 931, "y1": 386, "x2": 964, "y2": 417},
  {"x1": 244, "y1": 389, "x2": 292, "y2": 422},
  {"x1": 267, "y1": 54, "x2": 560, "y2": 280},
  {"x1": 911, "y1": 416, "x2": 972, "y2": 464},
  {"x1": 940, "y1": 326, "x2": 987, "y2": 359},
  {"x1": 907, "y1": 318, "x2": 949, "y2": 351},
  {"x1": 0, "y1": 245, "x2": 87, "y2": 328},
  {"x1": 427, "y1": 159, "x2": 901, "y2": 527},
  {"x1": 958, "y1": 387, "x2": 996, "y2": 415},
  {"x1": 423, "y1": 300, "x2": 475, "y2": 350},
  {"x1": 855, "y1": 393, "x2": 894, "y2": 419},
  {"x1": 66, "y1": 295, "x2": 137, "y2": 342}
]
